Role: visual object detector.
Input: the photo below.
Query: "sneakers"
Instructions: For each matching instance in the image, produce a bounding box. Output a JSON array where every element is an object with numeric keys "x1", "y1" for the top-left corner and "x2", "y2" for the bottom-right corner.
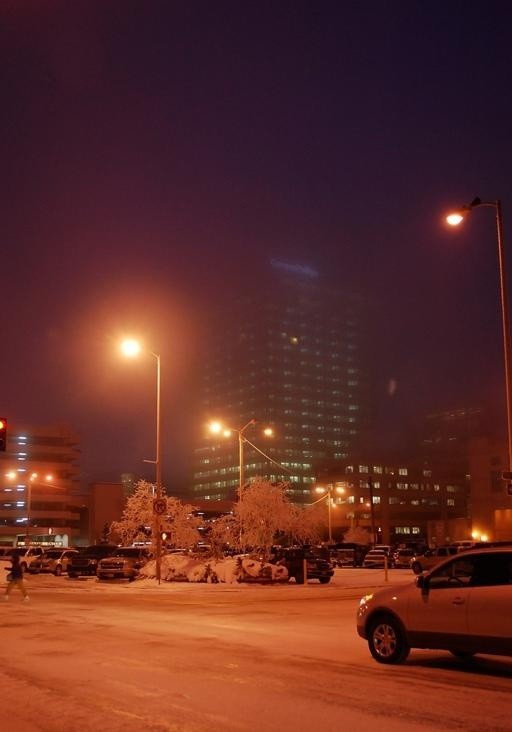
[
  {"x1": 3, "y1": 595, "x2": 8, "y2": 600},
  {"x1": 24, "y1": 597, "x2": 30, "y2": 601}
]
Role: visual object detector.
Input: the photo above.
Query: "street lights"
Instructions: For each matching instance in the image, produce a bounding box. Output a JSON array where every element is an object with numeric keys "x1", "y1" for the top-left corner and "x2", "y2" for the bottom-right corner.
[
  {"x1": 444, "y1": 201, "x2": 512, "y2": 473},
  {"x1": 6, "y1": 467, "x2": 55, "y2": 545},
  {"x1": 316, "y1": 481, "x2": 352, "y2": 541},
  {"x1": 120, "y1": 333, "x2": 163, "y2": 575},
  {"x1": 208, "y1": 416, "x2": 273, "y2": 540}
]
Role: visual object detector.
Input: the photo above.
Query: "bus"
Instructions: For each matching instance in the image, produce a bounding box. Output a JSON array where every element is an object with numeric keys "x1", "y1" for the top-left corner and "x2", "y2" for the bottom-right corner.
[{"x1": 13, "y1": 533, "x2": 56, "y2": 546}]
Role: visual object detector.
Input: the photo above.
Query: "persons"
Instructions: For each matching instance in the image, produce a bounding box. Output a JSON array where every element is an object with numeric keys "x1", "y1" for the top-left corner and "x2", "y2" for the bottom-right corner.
[{"x1": 1, "y1": 554, "x2": 30, "y2": 602}]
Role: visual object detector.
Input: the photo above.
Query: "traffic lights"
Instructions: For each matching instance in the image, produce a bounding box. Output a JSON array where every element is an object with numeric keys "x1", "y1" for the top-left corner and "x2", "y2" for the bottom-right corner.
[{"x1": 0, "y1": 417, "x2": 8, "y2": 455}]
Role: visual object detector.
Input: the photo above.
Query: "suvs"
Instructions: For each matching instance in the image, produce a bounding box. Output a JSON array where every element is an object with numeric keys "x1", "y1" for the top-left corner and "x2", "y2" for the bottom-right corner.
[
  {"x1": 269, "y1": 548, "x2": 335, "y2": 583},
  {"x1": 67, "y1": 544, "x2": 119, "y2": 578}
]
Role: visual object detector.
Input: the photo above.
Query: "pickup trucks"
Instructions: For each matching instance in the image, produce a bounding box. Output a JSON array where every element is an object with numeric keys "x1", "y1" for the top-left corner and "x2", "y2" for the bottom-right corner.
[{"x1": 409, "y1": 546, "x2": 471, "y2": 574}]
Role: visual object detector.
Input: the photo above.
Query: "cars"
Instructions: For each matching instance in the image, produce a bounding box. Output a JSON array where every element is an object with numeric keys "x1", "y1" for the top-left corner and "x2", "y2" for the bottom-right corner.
[
  {"x1": 357, "y1": 546, "x2": 512, "y2": 664},
  {"x1": 96, "y1": 547, "x2": 153, "y2": 579},
  {"x1": 1, "y1": 546, "x2": 45, "y2": 574},
  {"x1": 335, "y1": 543, "x2": 417, "y2": 568},
  {"x1": 140, "y1": 549, "x2": 290, "y2": 584},
  {"x1": 29, "y1": 547, "x2": 79, "y2": 576}
]
